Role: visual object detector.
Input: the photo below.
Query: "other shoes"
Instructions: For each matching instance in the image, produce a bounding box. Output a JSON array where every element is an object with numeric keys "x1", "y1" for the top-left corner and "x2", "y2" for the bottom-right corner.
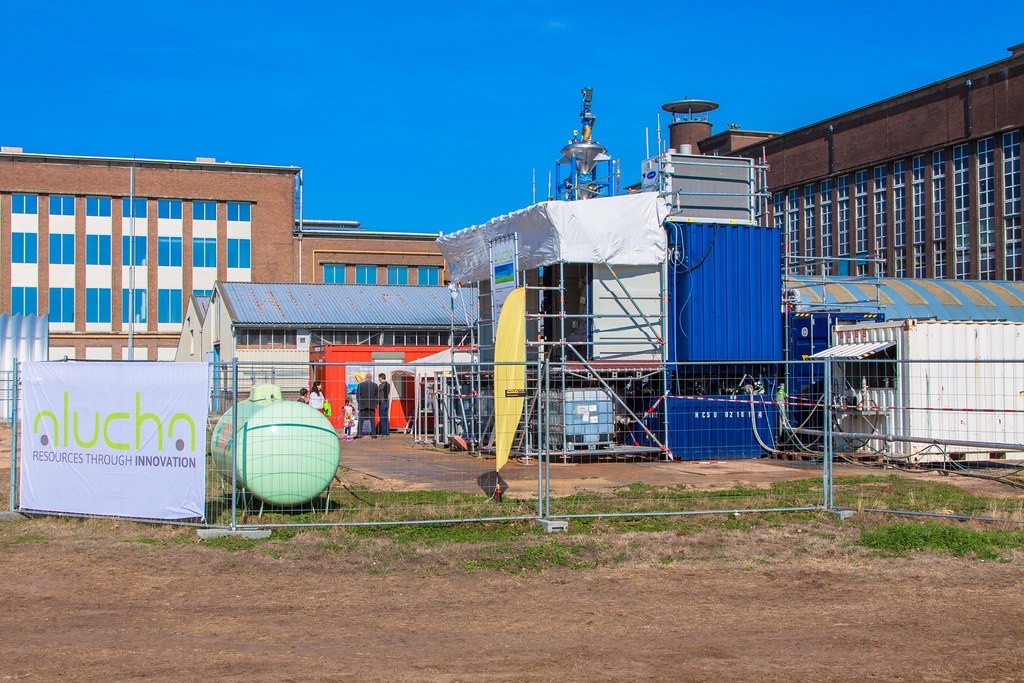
[
  {"x1": 353, "y1": 437, "x2": 359, "y2": 439},
  {"x1": 343, "y1": 435, "x2": 347, "y2": 439},
  {"x1": 347, "y1": 437, "x2": 351, "y2": 441},
  {"x1": 372, "y1": 436, "x2": 377, "y2": 438}
]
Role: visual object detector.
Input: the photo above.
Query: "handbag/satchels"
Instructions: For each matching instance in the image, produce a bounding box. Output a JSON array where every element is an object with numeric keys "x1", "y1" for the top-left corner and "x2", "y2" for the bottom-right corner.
[{"x1": 323, "y1": 399, "x2": 332, "y2": 416}]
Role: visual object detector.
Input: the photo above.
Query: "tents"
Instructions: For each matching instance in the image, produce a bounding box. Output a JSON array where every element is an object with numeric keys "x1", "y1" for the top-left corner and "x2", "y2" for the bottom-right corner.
[{"x1": 387, "y1": 348, "x2": 479, "y2": 441}]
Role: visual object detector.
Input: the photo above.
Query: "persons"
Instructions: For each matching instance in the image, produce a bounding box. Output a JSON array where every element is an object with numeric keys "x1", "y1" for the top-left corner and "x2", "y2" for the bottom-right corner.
[
  {"x1": 336, "y1": 396, "x2": 358, "y2": 442},
  {"x1": 309, "y1": 381, "x2": 325, "y2": 415},
  {"x1": 353, "y1": 373, "x2": 378, "y2": 439},
  {"x1": 377, "y1": 372, "x2": 391, "y2": 438},
  {"x1": 297, "y1": 387, "x2": 308, "y2": 403}
]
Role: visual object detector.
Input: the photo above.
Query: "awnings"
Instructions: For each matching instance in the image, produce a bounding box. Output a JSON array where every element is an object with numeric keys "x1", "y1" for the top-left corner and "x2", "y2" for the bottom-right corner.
[{"x1": 807, "y1": 342, "x2": 894, "y2": 360}]
